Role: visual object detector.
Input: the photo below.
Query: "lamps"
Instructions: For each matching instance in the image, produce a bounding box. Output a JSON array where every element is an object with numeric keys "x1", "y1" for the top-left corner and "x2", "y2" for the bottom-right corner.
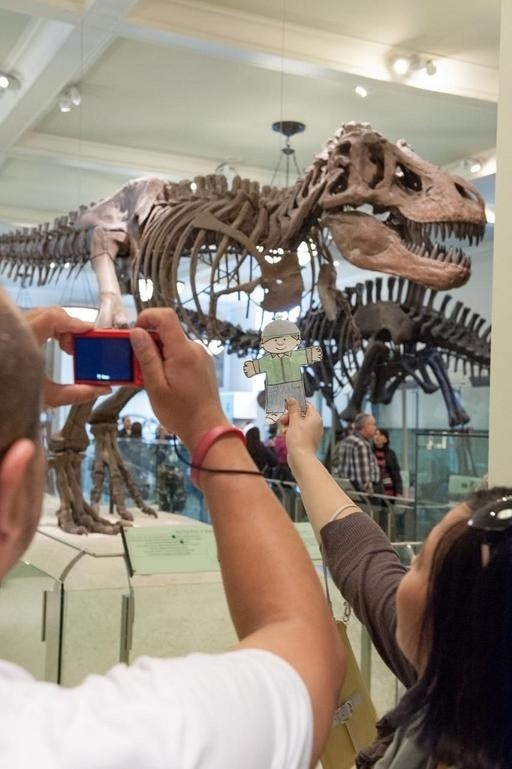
[
  {"x1": 59, "y1": 85, "x2": 82, "y2": 113},
  {"x1": 465, "y1": 157, "x2": 479, "y2": 173},
  {"x1": 425, "y1": 59, "x2": 437, "y2": 75}
]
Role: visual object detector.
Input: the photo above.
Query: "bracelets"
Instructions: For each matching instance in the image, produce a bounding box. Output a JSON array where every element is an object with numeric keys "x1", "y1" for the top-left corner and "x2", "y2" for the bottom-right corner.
[{"x1": 189, "y1": 423, "x2": 247, "y2": 490}]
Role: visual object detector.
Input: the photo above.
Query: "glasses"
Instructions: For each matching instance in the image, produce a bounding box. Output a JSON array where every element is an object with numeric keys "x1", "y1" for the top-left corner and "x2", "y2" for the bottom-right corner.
[{"x1": 466, "y1": 496, "x2": 512, "y2": 533}]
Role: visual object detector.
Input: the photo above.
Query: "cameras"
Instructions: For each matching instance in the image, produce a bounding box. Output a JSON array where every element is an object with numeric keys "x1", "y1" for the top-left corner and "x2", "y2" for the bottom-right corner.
[{"x1": 72, "y1": 329, "x2": 163, "y2": 388}]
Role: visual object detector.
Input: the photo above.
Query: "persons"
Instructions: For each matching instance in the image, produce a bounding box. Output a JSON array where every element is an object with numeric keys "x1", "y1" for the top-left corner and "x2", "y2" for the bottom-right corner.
[
  {"x1": 118, "y1": 415, "x2": 177, "y2": 465},
  {"x1": 0, "y1": 286, "x2": 353, "y2": 769},
  {"x1": 245, "y1": 412, "x2": 404, "y2": 508},
  {"x1": 273, "y1": 397, "x2": 512, "y2": 768}
]
